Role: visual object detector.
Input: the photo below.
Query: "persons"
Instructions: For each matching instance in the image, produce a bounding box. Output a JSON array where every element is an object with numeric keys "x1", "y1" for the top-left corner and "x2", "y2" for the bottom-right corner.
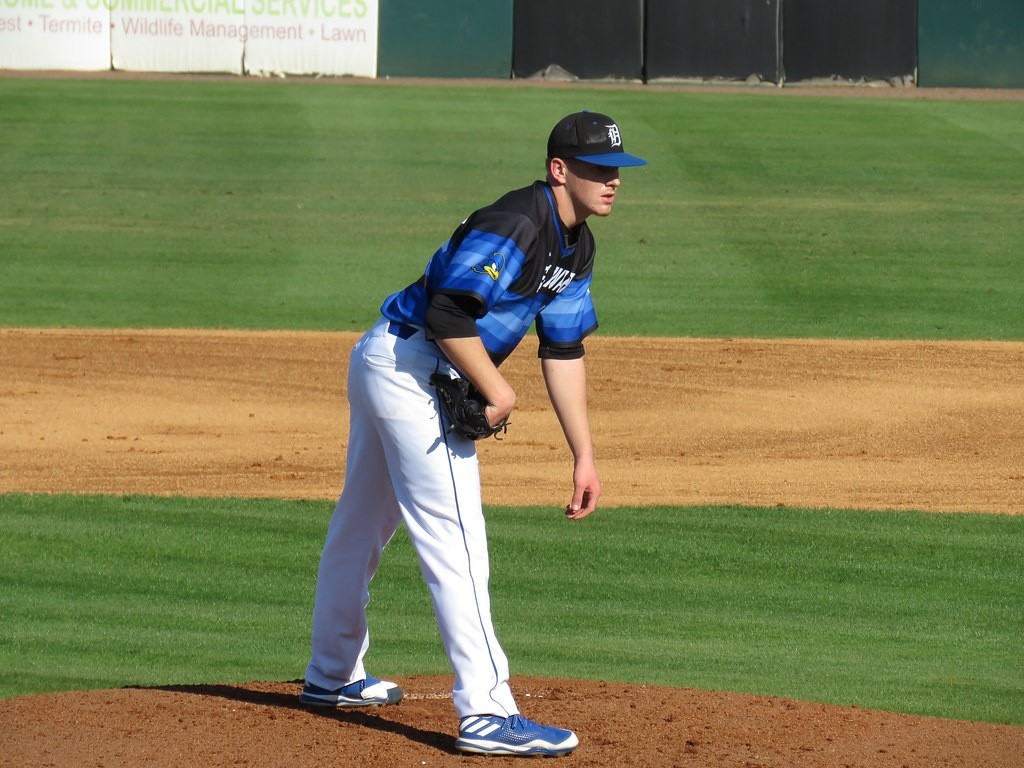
[{"x1": 299, "y1": 110, "x2": 647, "y2": 757}]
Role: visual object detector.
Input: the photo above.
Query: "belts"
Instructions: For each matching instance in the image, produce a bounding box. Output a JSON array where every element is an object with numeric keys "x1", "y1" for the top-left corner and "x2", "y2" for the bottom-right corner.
[{"x1": 389, "y1": 322, "x2": 417, "y2": 341}]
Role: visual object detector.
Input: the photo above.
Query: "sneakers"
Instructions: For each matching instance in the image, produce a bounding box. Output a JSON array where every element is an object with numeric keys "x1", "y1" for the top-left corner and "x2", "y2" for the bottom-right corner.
[
  {"x1": 455, "y1": 713, "x2": 580, "y2": 757},
  {"x1": 299, "y1": 673, "x2": 404, "y2": 706}
]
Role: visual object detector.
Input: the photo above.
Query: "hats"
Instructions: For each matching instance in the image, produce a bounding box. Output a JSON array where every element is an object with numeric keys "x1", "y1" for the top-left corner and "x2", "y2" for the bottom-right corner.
[{"x1": 547, "y1": 109, "x2": 648, "y2": 167}]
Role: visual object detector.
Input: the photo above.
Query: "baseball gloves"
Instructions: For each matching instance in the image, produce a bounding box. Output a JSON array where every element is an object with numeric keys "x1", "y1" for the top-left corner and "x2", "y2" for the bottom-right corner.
[{"x1": 433, "y1": 372, "x2": 510, "y2": 443}]
